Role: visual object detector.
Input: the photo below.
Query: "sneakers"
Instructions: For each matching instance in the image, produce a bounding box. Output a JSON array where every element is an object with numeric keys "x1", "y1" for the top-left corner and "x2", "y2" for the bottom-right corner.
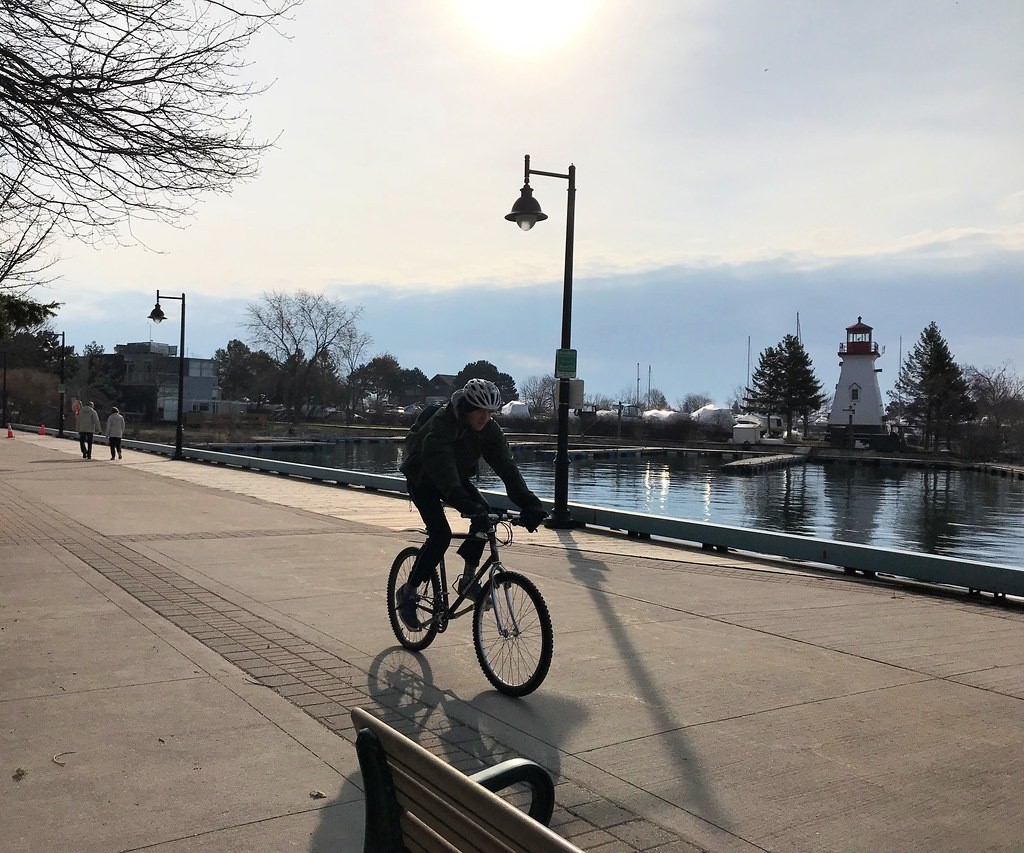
[
  {"x1": 396, "y1": 583, "x2": 420, "y2": 631},
  {"x1": 458, "y1": 578, "x2": 496, "y2": 607}
]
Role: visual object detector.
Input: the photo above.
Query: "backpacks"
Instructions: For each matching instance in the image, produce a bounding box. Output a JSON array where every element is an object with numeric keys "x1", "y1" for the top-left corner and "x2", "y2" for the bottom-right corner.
[{"x1": 404, "y1": 403, "x2": 464, "y2": 451}]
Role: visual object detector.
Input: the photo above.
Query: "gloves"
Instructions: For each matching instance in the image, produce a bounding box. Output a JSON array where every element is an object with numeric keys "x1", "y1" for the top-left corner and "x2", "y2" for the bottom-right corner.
[
  {"x1": 521, "y1": 505, "x2": 547, "y2": 534},
  {"x1": 466, "y1": 504, "x2": 490, "y2": 533}
]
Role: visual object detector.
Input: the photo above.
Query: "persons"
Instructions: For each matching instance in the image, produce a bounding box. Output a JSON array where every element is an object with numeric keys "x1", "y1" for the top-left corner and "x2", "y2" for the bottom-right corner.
[
  {"x1": 105, "y1": 406, "x2": 125, "y2": 460},
  {"x1": 76, "y1": 401, "x2": 102, "y2": 460},
  {"x1": 395, "y1": 379, "x2": 549, "y2": 631},
  {"x1": 73, "y1": 399, "x2": 81, "y2": 432}
]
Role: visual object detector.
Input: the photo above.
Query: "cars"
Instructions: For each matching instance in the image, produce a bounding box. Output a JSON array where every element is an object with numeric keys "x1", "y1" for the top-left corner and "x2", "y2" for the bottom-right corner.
[{"x1": 320, "y1": 401, "x2": 426, "y2": 424}]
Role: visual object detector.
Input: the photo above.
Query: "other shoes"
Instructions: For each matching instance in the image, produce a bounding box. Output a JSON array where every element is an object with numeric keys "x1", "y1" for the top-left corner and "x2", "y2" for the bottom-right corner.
[
  {"x1": 111, "y1": 458, "x2": 115, "y2": 460},
  {"x1": 118, "y1": 452, "x2": 123, "y2": 459},
  {"x1": 83, "y1": 453, "x2": 86, "y2": 458}
]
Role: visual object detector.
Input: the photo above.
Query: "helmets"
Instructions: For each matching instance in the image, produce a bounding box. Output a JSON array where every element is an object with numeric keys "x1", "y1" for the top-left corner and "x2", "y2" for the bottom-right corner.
[{"x1": 462, "y1": 378, "x2": 502, "y2": 410}]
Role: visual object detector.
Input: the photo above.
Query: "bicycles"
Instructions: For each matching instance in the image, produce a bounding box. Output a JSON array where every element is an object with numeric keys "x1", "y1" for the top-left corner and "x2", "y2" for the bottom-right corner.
[{"x1": 387, "y1": 509, "x2": 554, "y2": 698}]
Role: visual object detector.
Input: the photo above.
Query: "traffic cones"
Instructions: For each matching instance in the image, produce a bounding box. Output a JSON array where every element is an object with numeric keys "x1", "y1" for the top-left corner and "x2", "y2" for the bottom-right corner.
[
  {"x1": 6, "y1": 422, "x2": 15, "y2": 439},
  {"x1": 38, "y1": 424, "x2": 46, "y2": 435}
]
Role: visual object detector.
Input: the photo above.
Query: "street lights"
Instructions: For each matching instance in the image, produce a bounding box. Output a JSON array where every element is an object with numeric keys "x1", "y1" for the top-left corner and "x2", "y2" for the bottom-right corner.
[
  {"x1": 147, "y1": 290, "x2": 186, "y2": 460},
  {"x1": 505, "y1": 154, "x2": 577, "y2": 530},
  {"x1": 41, "y1": 331, "x2": 67, "y2": 440}
]
